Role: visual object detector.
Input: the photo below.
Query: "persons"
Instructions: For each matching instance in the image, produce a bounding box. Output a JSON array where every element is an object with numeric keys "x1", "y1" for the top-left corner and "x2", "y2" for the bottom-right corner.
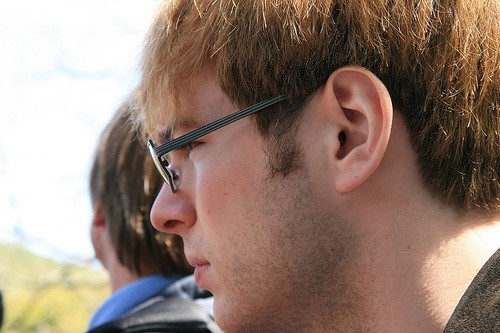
[
  {"x1": 136, "y1": 0, "x2": 500, "y2": 333},
  {"x1": 85, "y1": 89, "x2": 221, "y2": 333}
]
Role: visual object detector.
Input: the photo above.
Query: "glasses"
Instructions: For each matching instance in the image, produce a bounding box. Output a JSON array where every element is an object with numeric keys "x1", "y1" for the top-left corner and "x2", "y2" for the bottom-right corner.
[{"x1": 147, "y1": 92, "x2": 291, "y2": 193}]
